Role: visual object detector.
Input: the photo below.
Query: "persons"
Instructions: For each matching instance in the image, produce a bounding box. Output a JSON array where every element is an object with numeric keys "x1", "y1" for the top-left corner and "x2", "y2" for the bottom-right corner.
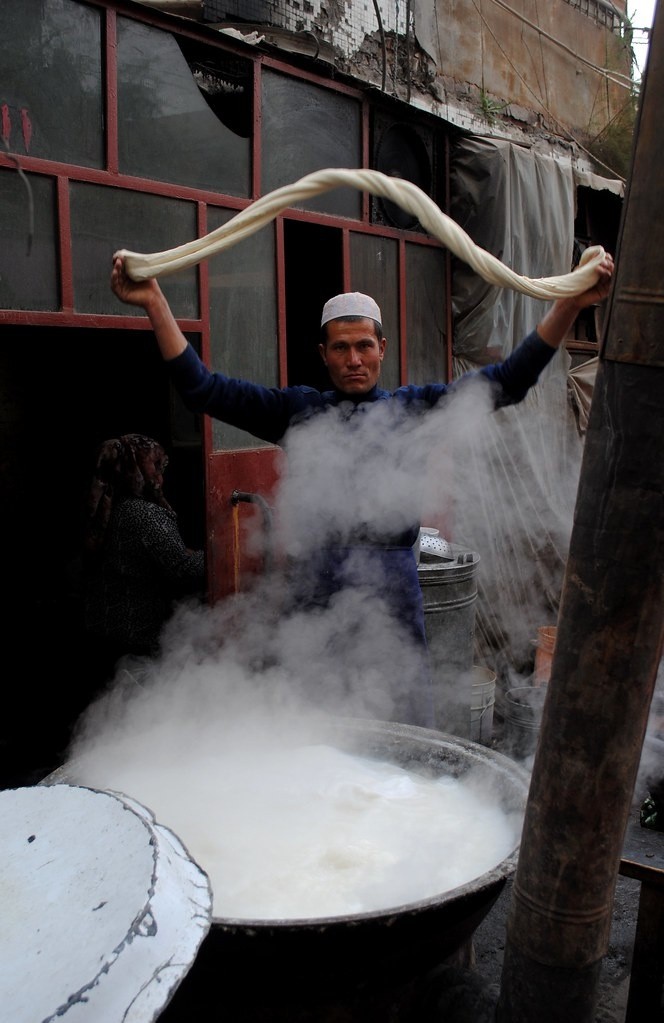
[
  {"x1": 108, "y1": 245, "x2": 616, "y2": 730},
  {"x1": 90, "y1": 433, "x2": 208, "y2": 659}
]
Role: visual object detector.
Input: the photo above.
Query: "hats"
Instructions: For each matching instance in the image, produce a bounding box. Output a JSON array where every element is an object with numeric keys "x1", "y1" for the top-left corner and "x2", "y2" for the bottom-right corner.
[{"x1": 320, "y1": 292, "x2": 382, "y2": 326}]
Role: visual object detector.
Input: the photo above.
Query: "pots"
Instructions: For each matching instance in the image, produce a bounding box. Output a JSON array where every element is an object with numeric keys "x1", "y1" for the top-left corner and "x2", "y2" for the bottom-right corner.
[{"x1": 36, "y1": 718, "x2": 534, "y2": 980}]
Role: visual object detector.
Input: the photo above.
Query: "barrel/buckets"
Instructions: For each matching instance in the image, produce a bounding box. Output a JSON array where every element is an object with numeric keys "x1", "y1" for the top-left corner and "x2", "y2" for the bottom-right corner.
[
  {"x1": 505, "y1": 687, "x2": 547, "y2": 762},
  {"x1": 416, "y1": 541, "x2": 480, "y2": 742},
  {"x1": 468, "y1": 666, "x2": 497, "y2": 749},
  {"x1": 532, "y1": 626, "x2": 558, "y2": 687}
]
